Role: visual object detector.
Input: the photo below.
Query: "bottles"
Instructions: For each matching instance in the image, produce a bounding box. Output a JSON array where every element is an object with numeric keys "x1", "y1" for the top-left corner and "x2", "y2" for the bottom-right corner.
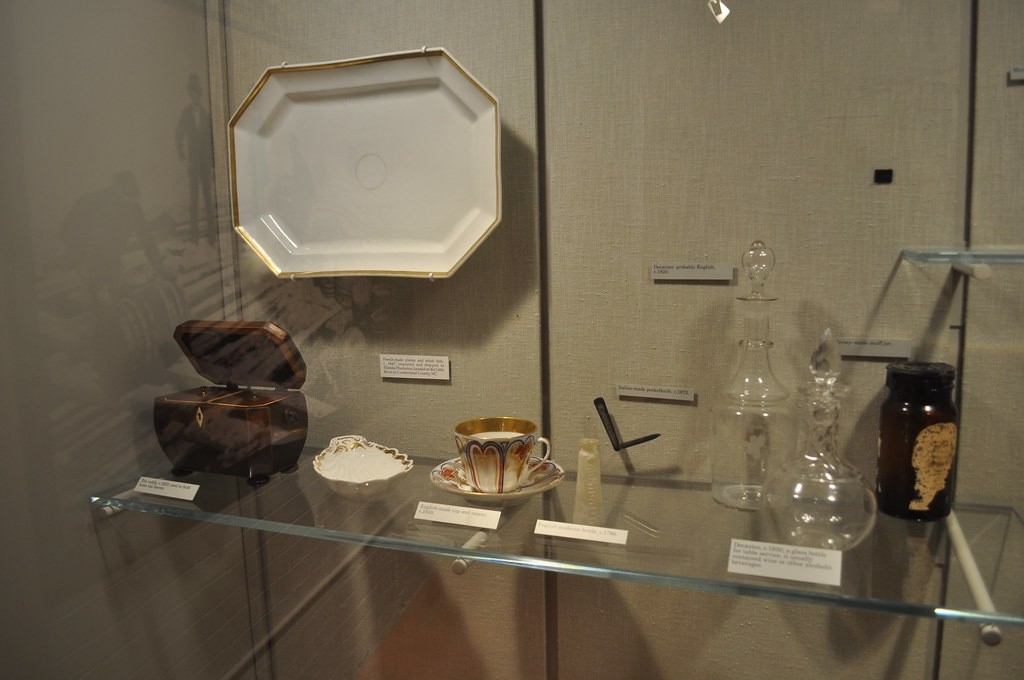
[
  {"x1": 875, "y1": 358, "x2": 961, "y2": 524},
  {"x1": 763, "y1": 328, "x2": 877, "y2": 556},
  {"x1": 709, "y1": 239, "x2": 802, "y2": 515}
]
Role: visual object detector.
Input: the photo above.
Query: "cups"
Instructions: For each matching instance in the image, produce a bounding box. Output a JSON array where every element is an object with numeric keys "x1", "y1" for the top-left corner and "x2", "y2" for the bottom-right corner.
[{"x1": 451, "y1": 416, "x2": 550, "y2": 493}]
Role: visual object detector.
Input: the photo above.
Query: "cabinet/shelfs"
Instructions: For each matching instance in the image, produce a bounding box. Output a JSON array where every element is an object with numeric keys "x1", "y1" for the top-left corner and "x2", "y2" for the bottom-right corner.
[{"x1": 89, "y1": 243, "x2": 1024, "y2": 649}]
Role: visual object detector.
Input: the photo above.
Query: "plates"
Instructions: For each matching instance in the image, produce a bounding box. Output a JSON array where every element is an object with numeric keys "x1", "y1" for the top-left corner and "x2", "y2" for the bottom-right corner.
[
  {"x1": 227, "y1": 46, "x2": 502, "y2": 283},
  {"x1": 313, "y1": 434, "x2": 414, "y2": 505},
  {"x1": 429, "y1": 456, "x2": 566, "y2": 510}
]
